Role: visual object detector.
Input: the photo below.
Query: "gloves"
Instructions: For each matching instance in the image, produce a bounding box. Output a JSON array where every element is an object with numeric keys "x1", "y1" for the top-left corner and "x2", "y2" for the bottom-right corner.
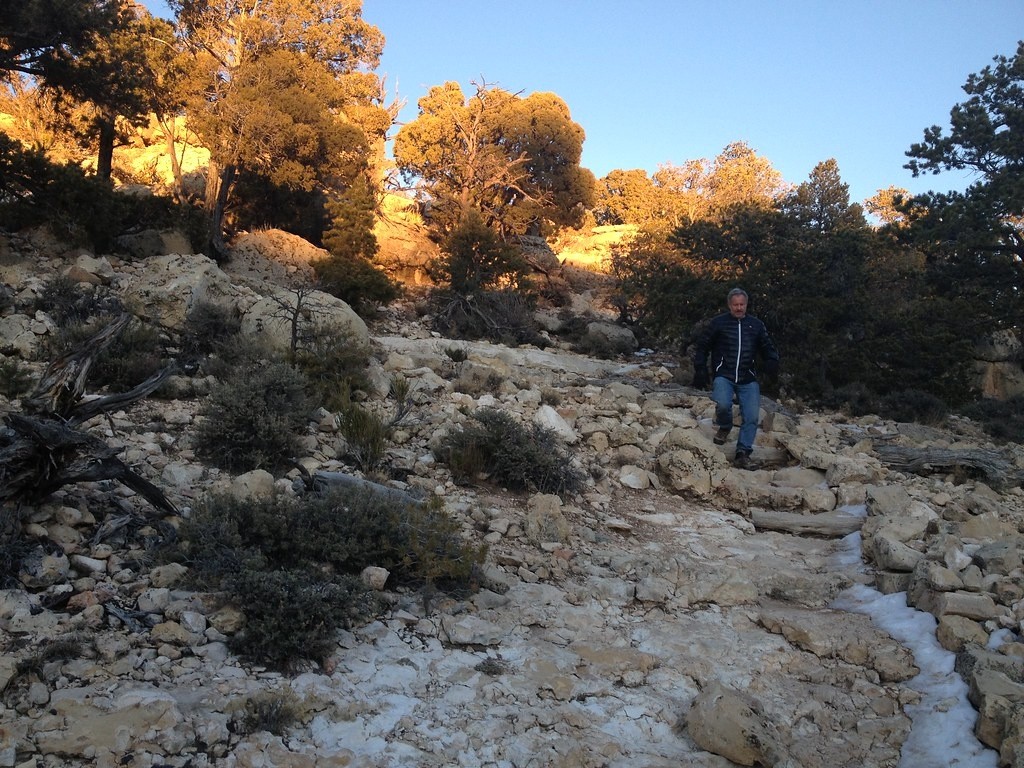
[
  {"x1": 693, "y1": 367, "x2": 711, "y2": 390},
  {"x1": 761, "y1": 361, "x2": 778, "y2": 385}
]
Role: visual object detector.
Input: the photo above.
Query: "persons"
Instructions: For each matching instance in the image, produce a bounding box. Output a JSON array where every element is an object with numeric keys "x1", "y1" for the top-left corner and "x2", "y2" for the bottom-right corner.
[{"x1": 694, "y1": 288, "x2": 778, "y2": 471}]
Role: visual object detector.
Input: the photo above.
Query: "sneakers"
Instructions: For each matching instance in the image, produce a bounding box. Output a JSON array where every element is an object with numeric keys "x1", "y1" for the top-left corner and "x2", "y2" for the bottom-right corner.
[
  {"x1": 713, "y1": 427, "x2": 732, "y2": 445},
  {"x1": 733, "y1": 448, "x2": 759, "y2": 471}
]
